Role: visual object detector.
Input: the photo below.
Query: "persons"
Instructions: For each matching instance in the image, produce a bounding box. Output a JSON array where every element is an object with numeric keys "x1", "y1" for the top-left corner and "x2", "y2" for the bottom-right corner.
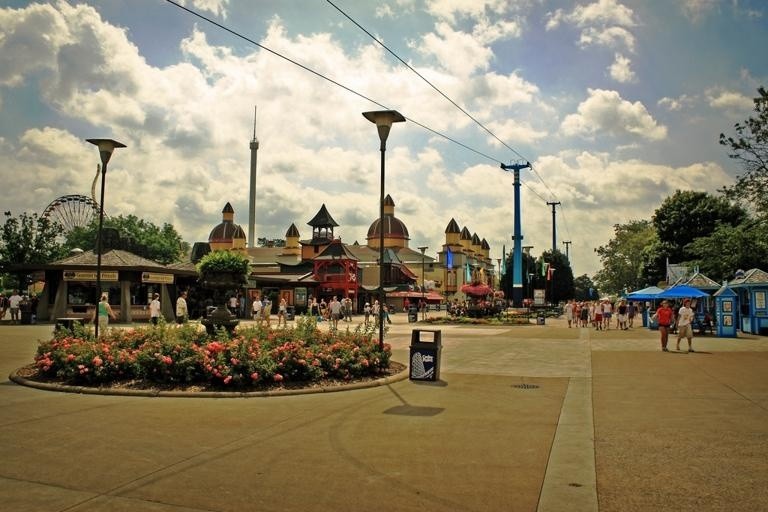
[
  {"x1": 0, "y1": 289, "x2": 38, "y2": 324},
  {"x1": 404, "y1": 297, "x2": 410, "y2": 311},
  {"x1": 418, "y1": 299, "x2": 506, "y2": 319},
  {"x1": 563, "y1": 298, "x2": 715, "y2": 337},
  {"x1": 652, "y1": 299, "x2": 674, "y2": 352},
  {"x1": 149, "y1": 292, "x2": 162, "y2": 329},
  {"x1": 676, "y1": 298, "x2": 695, "y2": 352},
  {"x1": 89, "y1": 294, "x2": 116, "y2": 334},
  {"x1": 228, "y1": 293, "x2": 390, "y2": 332},
  {"x1": 174, "y1": 290, "x2": 188, "y2": 326}
]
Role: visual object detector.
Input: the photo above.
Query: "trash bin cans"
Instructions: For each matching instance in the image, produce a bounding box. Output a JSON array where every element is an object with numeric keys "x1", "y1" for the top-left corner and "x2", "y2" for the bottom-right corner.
[
  {"x1": 408, "y1": 308, "x2": 417, "y2": 322},
  {"x1": 389, "y1": 305, "x2": 396, "y2": 314},
  {"x1": 286, "y1": 306, "x2": 296, "y2": 321},
  {"x1": 409, "y1": 329, "x2": 443, "y2": 381},
  {"x1": 21, "y1": 311, "x2": 32, "y2": 324},
  {"x1": 55, "y1": 318, "x2": 85, "y2": 331}
]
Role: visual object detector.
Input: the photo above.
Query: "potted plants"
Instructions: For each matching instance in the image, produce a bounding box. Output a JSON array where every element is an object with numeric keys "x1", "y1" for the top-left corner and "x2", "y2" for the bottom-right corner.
[{"x1": 196, "y1": 249, "x2": 253, "y2": 320}]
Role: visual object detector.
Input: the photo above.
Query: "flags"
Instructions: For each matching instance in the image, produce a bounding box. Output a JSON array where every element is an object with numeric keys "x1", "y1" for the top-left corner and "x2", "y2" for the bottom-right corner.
[
  {"x1": 541, "y1": 254, "x2": 546, "y2": 276},
  {"x1": 547, "y1": 265, "x2": 551, "y2": 281},
  {"x1": 446, "y1": 247, "x2": 453, "y2": 270},
  {"x1": 467, "y1": 262, "x2": 472, "y2": 284},
  {"x1": 501, "y1": 244, "x2": 506, "y2": 275}
]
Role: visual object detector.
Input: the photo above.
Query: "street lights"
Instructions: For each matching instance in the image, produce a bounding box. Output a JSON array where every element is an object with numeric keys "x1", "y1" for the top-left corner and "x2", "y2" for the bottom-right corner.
[
  {"x1": 522, "y1": 244, "x2": 534, "y2": 311},
  {"x1": 82, "y1": 137, "x2": 129, "y2": 337},
  {"x1": 360, "y1": 109, "x2": 409, "y2": 354},
  {"x1": 417, "y1": 246, "x2": 428, "y2": 320},
  {"x1": 495, "y1": 258, "x2": 502, "y2": 282}
]
